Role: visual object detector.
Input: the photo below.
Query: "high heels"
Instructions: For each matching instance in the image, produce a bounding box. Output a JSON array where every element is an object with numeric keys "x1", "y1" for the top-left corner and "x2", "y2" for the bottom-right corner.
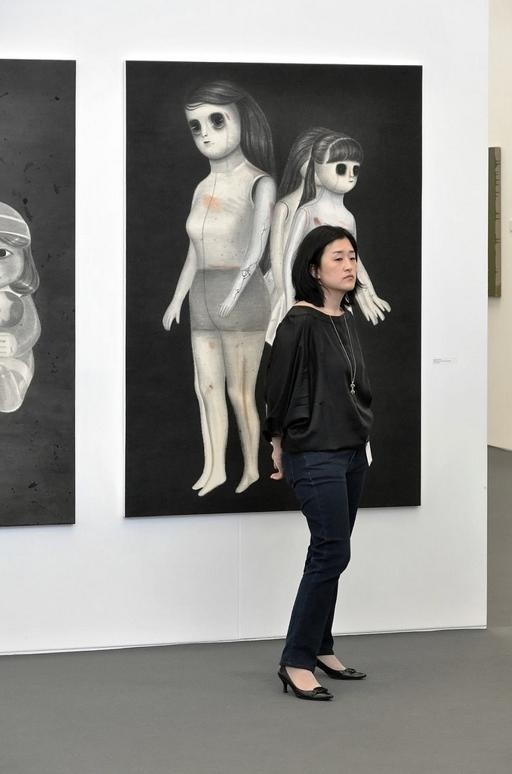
[
  {"x1": 277, "y1": 664, "x2": 334, "y2": 700},
  {"x1": 311, "y1": 652, "x2": 367, "y2": 681}
]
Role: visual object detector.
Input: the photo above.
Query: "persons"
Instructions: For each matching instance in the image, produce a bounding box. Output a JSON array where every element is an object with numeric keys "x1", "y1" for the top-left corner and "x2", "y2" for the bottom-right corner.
[
  {"x1": 255, "y1": 221, "x2": 377, "y2": 705},
  {"x1": 0, "y1": 198, "x2": 49, "y2": 421}
]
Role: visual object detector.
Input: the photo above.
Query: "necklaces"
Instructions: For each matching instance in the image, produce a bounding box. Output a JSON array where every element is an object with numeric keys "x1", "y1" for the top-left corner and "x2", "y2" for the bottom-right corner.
[{"x1": 327, "y1": 307, "x2": 361, "y2": 401}]
